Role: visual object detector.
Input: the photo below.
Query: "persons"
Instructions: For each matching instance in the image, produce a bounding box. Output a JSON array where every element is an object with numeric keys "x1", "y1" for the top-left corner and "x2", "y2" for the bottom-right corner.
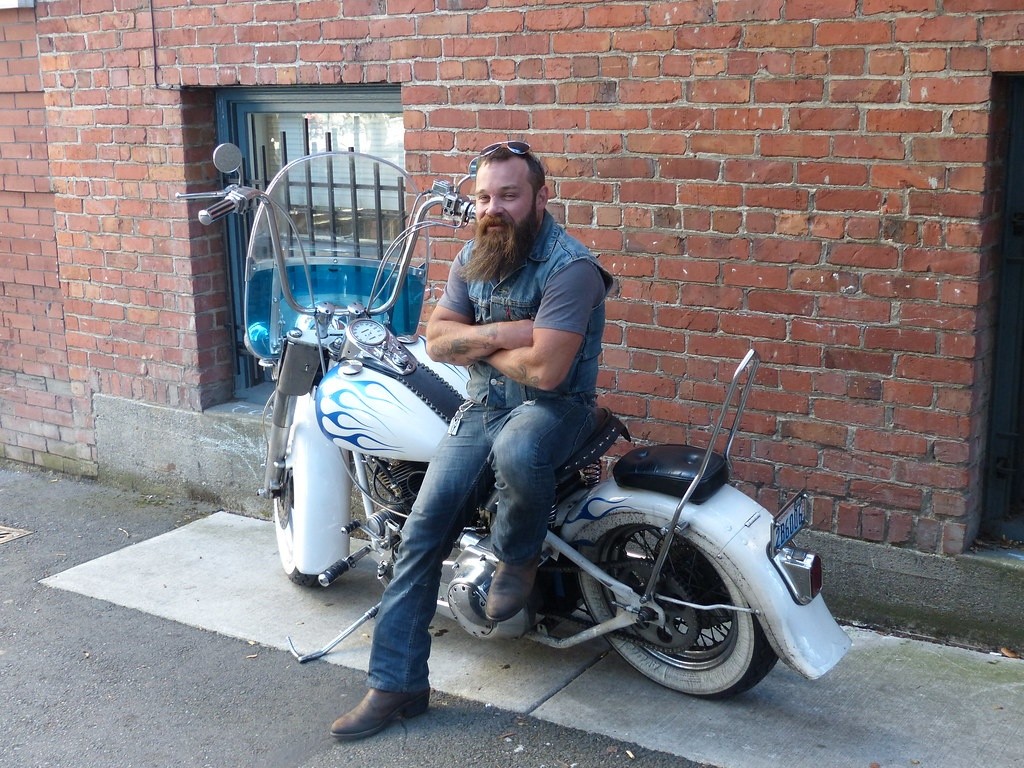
[{"x1": 324, "y1": 141, "x2": 613, "y2": 738}]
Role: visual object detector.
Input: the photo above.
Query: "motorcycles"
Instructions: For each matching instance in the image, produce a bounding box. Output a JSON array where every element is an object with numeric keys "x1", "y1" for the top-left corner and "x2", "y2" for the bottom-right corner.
[{"x1": 175, "y1": 139, "x2": 856, "y2": 701}]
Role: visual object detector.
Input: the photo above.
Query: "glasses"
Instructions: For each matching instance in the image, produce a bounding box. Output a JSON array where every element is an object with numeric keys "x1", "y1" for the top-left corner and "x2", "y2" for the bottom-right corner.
[{"x1": 478, "y1": 140, "x2": 539, "y2": 166}]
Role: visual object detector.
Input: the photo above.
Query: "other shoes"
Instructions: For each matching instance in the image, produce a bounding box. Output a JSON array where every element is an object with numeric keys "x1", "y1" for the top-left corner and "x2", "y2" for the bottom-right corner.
[
  {"x1": 329, "y1": 681, "x2": 430, "y2": 739},
  {"x1": 486, "y1": 553, "x2": 538, "y2": 622}
]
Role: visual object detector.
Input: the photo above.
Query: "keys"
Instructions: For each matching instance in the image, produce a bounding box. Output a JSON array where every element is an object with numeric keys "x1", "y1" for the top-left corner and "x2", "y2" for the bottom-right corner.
[{"x1": 447, "y1": 410, "x2": 464, "y2": 436}]
